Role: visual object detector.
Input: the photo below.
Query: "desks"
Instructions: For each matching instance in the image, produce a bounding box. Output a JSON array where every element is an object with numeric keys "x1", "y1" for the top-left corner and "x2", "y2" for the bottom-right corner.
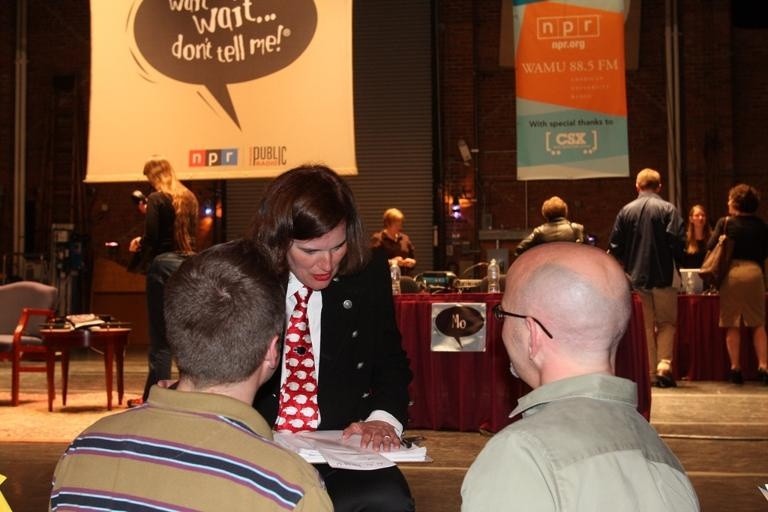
[
  {"x1": 675, "y1": 293, "x2": 767, "y2": 382},
  {"x1": 37, "y1": 327, "x2": 133, "y2": 411},
  {"x1": 391, "y1": 293, "x2": 652, "y2": 436}
]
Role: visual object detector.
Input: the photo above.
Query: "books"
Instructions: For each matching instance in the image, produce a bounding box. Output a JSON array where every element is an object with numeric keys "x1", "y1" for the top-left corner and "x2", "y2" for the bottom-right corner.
[{"x1": 273, "y1": 429, "x2": 427, "y2": 470}]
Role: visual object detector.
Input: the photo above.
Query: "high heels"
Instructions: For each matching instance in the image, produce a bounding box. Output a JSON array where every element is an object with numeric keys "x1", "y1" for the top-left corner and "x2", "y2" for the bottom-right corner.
[
  {"x1": 724, "y1": 368, "x2": 740, "y2": 383},
  {"x1": 757, "y1": 368, "x2": 768, "y2": 384}
]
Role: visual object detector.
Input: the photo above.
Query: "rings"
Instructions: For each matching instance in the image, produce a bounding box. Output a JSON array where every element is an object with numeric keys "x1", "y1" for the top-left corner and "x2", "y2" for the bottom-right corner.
[{"x1": 383, "y1": 435, "x2": 391, "y2": 439}]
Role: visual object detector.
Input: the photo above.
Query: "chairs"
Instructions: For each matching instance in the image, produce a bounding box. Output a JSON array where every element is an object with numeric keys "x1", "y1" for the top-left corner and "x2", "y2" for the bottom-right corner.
[{"x1": 0, "y1": 282, "x2": 59, "y2": 407}]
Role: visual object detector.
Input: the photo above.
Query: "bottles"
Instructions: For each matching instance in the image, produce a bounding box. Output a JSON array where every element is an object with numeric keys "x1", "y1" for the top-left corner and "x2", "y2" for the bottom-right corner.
[
  {"x1": 389, "y1": 260, "x2": 400, "y2": 294},
  {"x1": 487, "y1": 259, "x2": 500, "y2": 293}
]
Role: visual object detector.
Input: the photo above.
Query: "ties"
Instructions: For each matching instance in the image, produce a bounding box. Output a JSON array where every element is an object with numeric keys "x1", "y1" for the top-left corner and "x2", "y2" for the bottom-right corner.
[{"x1": 271, "y1": 284, "x2": 318, "y2": 433}]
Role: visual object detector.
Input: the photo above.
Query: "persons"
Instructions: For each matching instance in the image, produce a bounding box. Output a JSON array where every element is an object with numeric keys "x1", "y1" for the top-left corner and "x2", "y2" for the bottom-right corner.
[
  {"x1": 676, "y1": 204, "x2": 711, "y2": 269},
  {"x1": 127, "y1": 153, "x2": 199, "y2": 409},
  {"x1": 706, "y1": 184, "x2": 768, "y2": 384},
  {"x1": 245, "y1": 164, "x2": 414, "y2": 512},
  {"x1": 368, "y1": 208, "x2": 419, "y2": 276},
  {"x1": 459, "y1": 241, "x2": 700, "y2": 511},
  {"x1": 608, "y1": 168, "x2": 686, "y2": 387},
  {"x1": 514, "y1": 196, "x2": 584, "y2": 256},
  {"x1": 48, "y1": 242, "x2": 334, "y2": 512}
]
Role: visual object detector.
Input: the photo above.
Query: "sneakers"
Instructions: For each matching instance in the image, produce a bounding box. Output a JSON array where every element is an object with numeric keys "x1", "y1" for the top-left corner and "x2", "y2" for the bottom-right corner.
[{"x1": 125, "y1": 398, "x2": 142, "y2": 406}]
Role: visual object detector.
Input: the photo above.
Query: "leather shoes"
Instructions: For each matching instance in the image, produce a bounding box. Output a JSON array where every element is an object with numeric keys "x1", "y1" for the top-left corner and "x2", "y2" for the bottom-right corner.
[
  {"x1": 651, "y1": 382, "x2": 661, "y2": 388},
  {"x1": 656, "y1": 368, "x2": 677, "y2": 387}
]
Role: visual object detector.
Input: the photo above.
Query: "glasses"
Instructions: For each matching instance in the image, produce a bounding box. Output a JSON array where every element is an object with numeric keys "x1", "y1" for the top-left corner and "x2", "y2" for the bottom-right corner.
[{"x1": 492, "y1": 302, "x2": 553, "y2": 339}]
